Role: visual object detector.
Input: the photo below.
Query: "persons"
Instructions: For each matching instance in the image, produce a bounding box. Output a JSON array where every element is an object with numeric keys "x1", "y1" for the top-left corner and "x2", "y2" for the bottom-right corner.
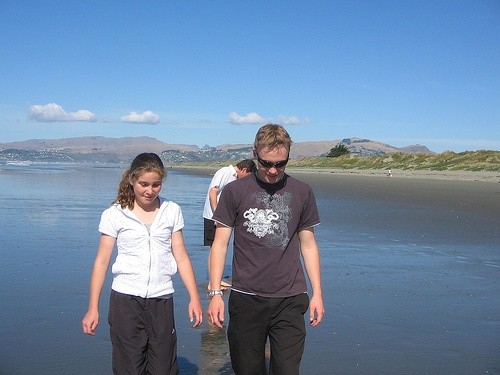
[
  {"x1": 205, "y1": 124, "x2": 325, "y2": 375},
  {"x1": 81, "y1": 153, "x2": 203, "y2": 375},
  {"x1": 202, "y1": 159, "x2": 258, "y2": 292}
]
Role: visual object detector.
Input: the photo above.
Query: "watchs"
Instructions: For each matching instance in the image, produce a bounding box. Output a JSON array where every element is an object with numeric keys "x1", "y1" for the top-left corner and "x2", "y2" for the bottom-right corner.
[{"x1": 206, "y1": 290, "x2": 223, "y2": 299}]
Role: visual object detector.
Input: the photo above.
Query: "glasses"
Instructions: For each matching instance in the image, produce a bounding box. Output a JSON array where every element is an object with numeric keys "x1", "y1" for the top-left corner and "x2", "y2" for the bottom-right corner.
[{"x1": 256, "y1": 150, "x2": 289, "y2": 168}]
